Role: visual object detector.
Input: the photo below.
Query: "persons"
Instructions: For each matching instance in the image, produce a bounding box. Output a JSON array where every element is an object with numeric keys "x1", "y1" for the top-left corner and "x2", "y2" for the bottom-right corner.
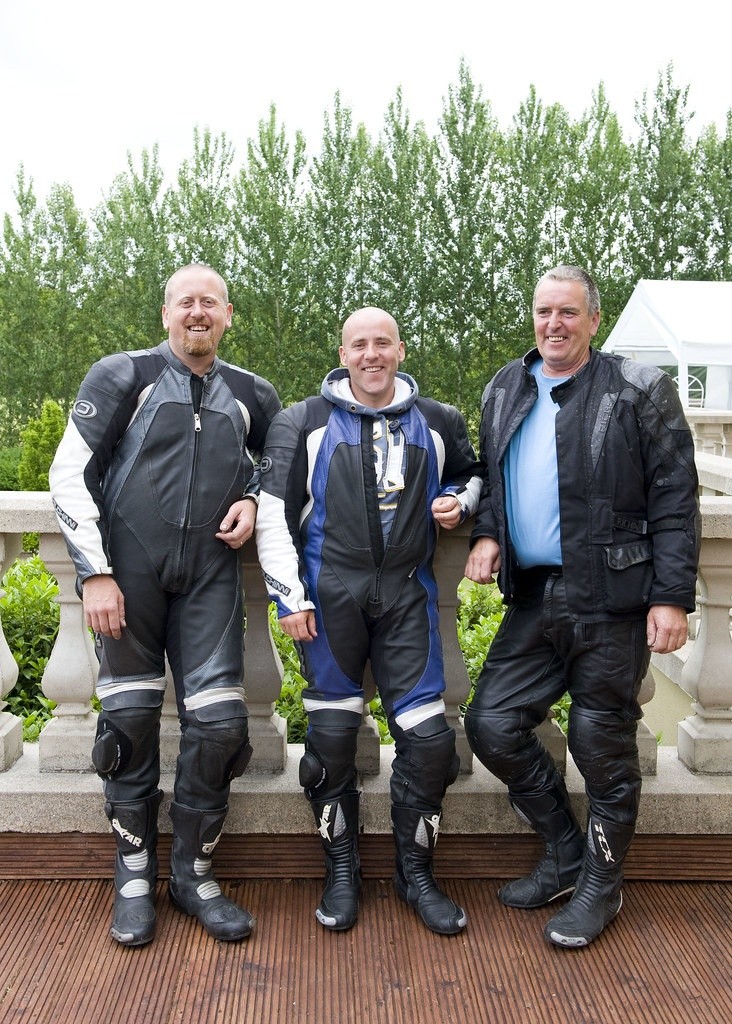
[
  {"x1": 255, "y1": 307, "x2": 483, "y2": 934},
  {"x1": 49, "y1": 264, "x2": 284, "y2": 946},
  {"x1": 464, "y1": 265, "x2": 701, "y2": 947}
]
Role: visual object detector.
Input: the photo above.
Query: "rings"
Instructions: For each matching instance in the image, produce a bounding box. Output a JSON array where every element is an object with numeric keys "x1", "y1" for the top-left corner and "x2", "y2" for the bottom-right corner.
[{"x1": 240, "y1": 540, "x2": 243, "y2": 545}]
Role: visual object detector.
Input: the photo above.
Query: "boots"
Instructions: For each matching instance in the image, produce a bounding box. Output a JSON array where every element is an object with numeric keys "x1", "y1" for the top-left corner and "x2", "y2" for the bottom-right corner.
[
  {"x1": 309, "y1": 789, "x2": 363, "y2": 931},
  {"x1": 542, "y1": 807, "x2": 637, "y2": 949},
  {"x1": 497, "y1": 772, "x2": 587, "y2": 908},
  {"x1": 165, "y1": 799, "x2": 256, "y2": 940},
  {"x1": 390, "y1": 802, "x2": 467, "y2": 934},
  {"x1": 104, "y1": 787, "x2": 164, "y2": 945}
]
